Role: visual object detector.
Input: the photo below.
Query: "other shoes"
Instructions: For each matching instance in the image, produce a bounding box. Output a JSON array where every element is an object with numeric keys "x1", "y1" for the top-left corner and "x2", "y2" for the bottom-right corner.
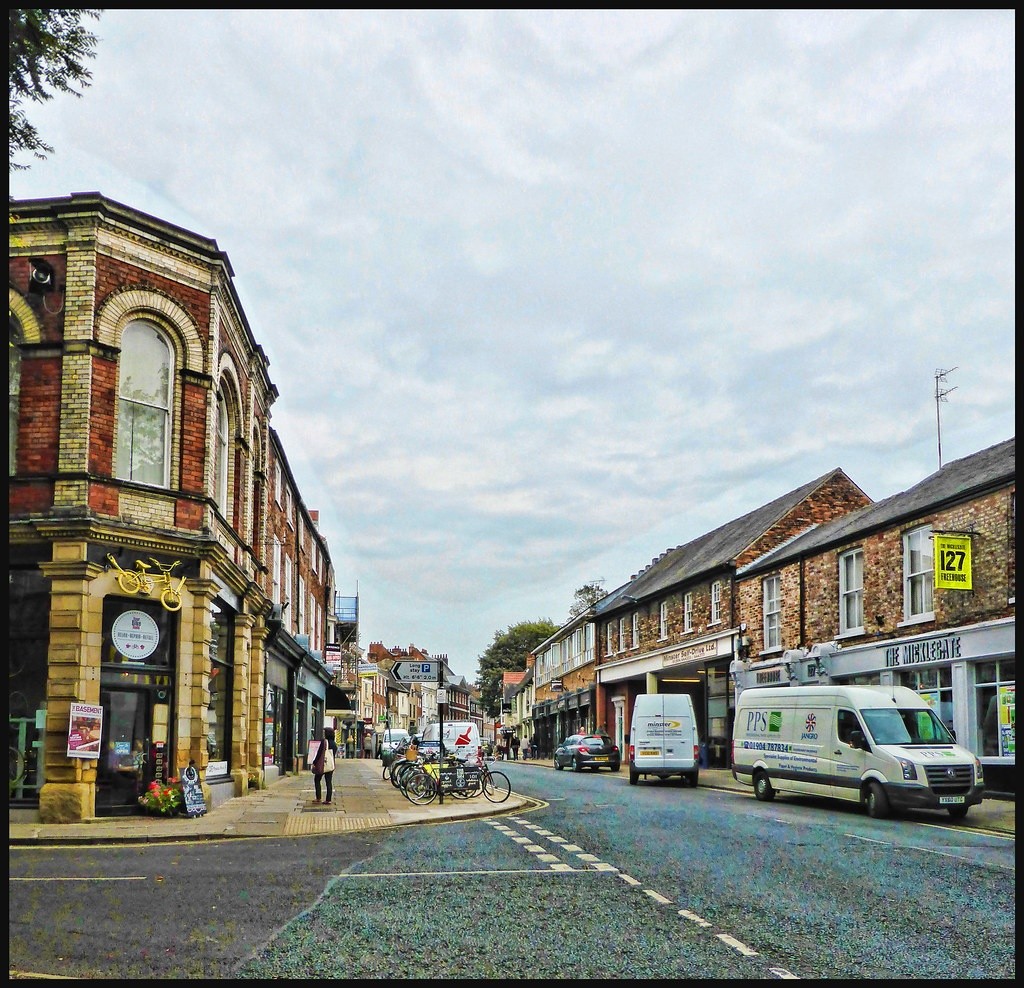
[
  {"x1": 312, "y1": 798, "x2": 321, "y2": 802},
  {"x1": 322, "y1": 800, "x2": 331, "y2": 804}
]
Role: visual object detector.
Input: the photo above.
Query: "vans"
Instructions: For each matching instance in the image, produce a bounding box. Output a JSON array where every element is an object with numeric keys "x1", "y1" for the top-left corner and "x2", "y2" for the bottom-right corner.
[
  {"x1": 421, "y1": 720, "x2": 484, "y2": 763},
  {"x1": 730, "y1": 684, "x2": 986, "y2": 819},
  {"x1": 624, "y1": 693, "x2": 700, "y2": 788},
  {"x1": 379, "y1": 728, "x2": 411, "y2": 756}
]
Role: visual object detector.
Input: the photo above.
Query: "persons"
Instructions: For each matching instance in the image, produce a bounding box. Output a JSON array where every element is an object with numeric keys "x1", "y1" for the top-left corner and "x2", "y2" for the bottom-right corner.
[
  {"x1": 511, "y1": 737, "x2": 520, "y2": 761},
  {"x1": 521, "y1": 735, "x2": 530, "y2": 760},
  {"x1": 363, "y1": 733, "x2": 372, "y2": 758},
  {"x1": 312, "y1": 727, "x2": 338, "y2": 804},
  {"x1": 529, "y1": 734, "x2": 539, "y2": 760}
]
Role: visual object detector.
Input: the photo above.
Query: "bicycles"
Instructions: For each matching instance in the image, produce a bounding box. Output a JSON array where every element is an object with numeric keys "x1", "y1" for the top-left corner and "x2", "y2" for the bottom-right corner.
[{"x1": 382, "y1": 745, "x2": 512, "y2": 806}]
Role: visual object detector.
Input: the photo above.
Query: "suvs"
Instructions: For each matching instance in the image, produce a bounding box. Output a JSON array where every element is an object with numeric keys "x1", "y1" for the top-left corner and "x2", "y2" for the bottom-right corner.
[{"x1": 416, "y1": 740, "x2": 447, "y2": 759}]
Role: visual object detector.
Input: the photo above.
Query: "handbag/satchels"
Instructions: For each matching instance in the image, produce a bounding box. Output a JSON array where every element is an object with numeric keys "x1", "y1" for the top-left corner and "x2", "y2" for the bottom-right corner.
[
  {"x1": 311, "y1": 740, "x2": 327, "y2": 775},
  {"x1": 324, "y1": 739, "x2": 335, "y2": 773}
]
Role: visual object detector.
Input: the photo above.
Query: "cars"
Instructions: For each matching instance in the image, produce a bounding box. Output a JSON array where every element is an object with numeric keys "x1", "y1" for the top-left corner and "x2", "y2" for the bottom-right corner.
[
  {"x1": 207, "y1": 732, "x2": 217, "y2": 759},
  {"x1": 553, "y1": 734, "x2": 621, "y2": 772}
]
